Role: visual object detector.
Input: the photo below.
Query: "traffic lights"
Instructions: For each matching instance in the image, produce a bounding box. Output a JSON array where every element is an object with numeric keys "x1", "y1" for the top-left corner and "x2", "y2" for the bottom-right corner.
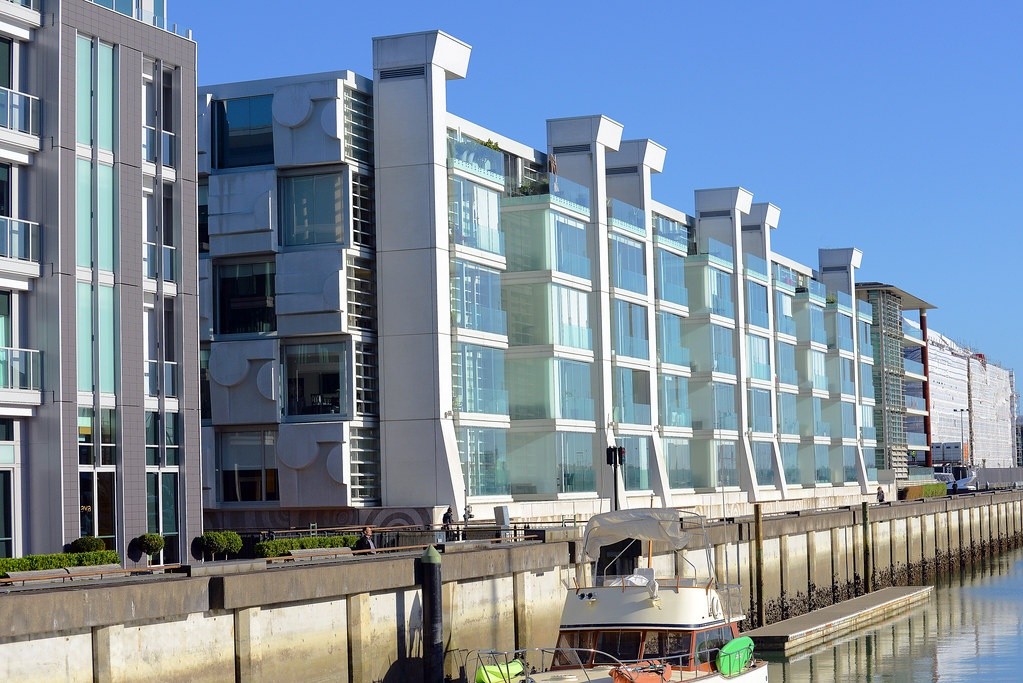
[{"x1": 618, "y1": 446, "x2": 626, "y2": 466}]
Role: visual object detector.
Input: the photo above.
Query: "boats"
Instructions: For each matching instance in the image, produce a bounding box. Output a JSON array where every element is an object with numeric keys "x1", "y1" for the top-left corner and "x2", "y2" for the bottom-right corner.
[
  {"x1": 474, "y1": 657, "x2": 524, "y2": 683},
  {"x1": 421, "y1": 495, "x2": 768, "y2": 683},
  {"x1": 609, "y1": 658, "x2": 672, "y2": 683}
]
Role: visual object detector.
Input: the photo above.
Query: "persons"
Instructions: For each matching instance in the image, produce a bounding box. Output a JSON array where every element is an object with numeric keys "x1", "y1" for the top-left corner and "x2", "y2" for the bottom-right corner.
[
  {"x1": 952, "y1": 481, "x2": 958, "y2": 494},
  {"x1": 356, "y1": 528, "x2": 377, "y2": 555},
  {"x1": 443, "y1": 507, "x2": 454, "y2": 529},
  {"x1": 877, "y1": 487, "x2": 884, "y2": 502}
]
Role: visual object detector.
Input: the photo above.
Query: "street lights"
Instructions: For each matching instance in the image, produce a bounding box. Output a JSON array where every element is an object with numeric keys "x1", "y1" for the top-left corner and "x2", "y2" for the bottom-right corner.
[{"x1": 954, "y1": 408, "x2": 969, "y2": 466}]
